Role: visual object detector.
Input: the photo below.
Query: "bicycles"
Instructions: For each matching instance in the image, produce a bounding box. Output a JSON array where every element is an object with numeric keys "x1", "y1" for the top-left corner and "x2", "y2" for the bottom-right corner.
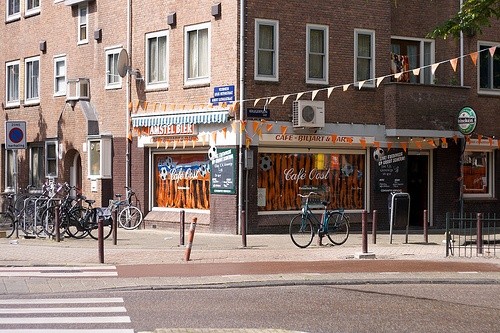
[
  {"x1": 289, "y1": 192, "x2": 350, "y2": 249},
  {"x1": 0, "y1": 176, "x2": 113, "y2": 240},
  {"x1": 104, "y1": 187, "x2": 143, "y2": 230}
]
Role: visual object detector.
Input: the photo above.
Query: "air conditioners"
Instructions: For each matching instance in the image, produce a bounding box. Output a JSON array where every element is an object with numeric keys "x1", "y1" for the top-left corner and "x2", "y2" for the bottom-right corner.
[{"x1": 293, "y1": 100, "x2": 325, "y2": 128}]
